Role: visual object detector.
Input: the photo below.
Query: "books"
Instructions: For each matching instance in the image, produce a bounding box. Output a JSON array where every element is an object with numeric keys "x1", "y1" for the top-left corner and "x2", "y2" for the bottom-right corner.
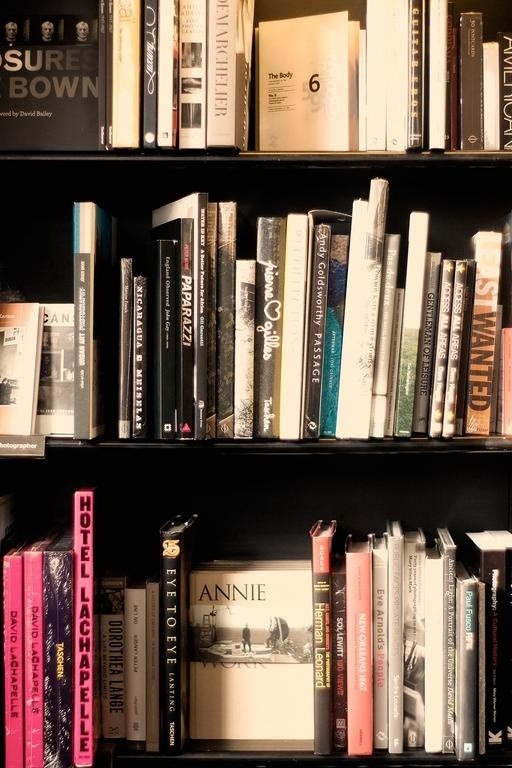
[
  {"x1": 0, "y1": 177, "x2": 512, "y2": 440},
  {"x1": 0, "y1": 0, "x2": 512, "y2": 154},
  {"x1": 0, "y1": 487, "x2": 512, "y2": 768}
]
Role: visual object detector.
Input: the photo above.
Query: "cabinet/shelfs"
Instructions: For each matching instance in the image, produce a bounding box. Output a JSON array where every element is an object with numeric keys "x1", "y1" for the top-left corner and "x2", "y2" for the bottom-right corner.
[{"x1": 2, "y1": 0, "x2": 511, "y2": 767}]
